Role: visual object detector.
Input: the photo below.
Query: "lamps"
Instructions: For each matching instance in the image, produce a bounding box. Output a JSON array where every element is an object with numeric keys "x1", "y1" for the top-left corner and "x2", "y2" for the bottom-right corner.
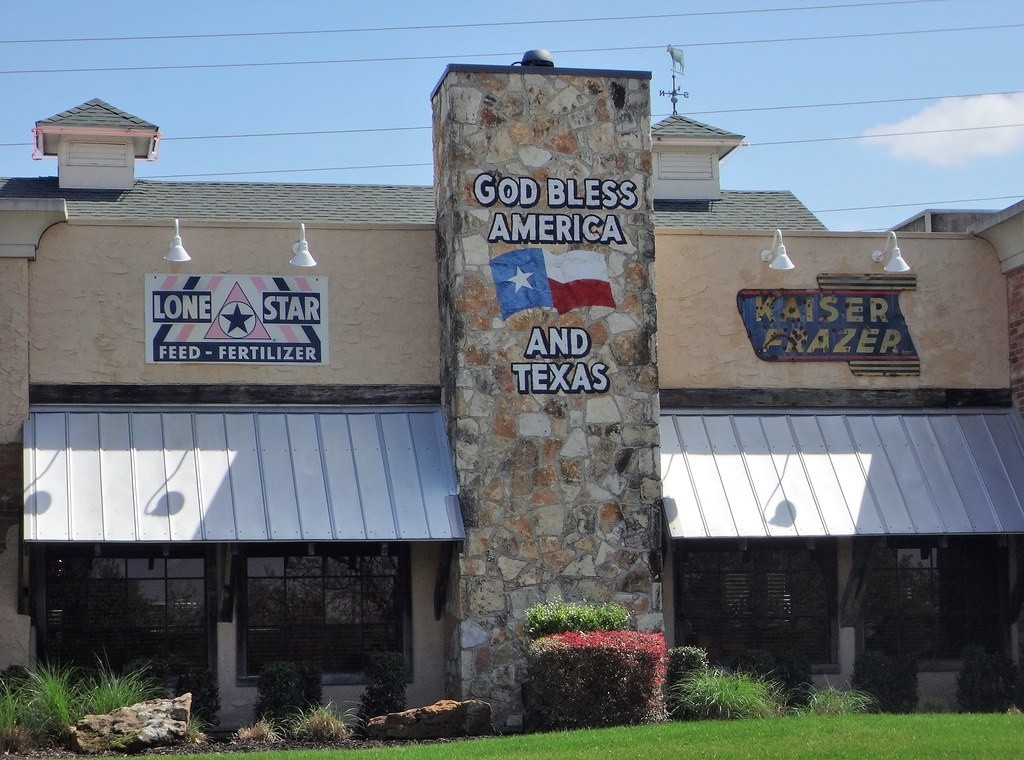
[
  {"x1": 163, "y1": 218, "x2": 192, "y2": 262},
  {"x1": 289, "y1": 223, "x2": 318, "y2": 267},
  {"x1": 872, "y1": 231, "x2": 911, "y2": 274},
  {"x1": 761, "y1": 229, "x2": 795, "y2": 270}
]
